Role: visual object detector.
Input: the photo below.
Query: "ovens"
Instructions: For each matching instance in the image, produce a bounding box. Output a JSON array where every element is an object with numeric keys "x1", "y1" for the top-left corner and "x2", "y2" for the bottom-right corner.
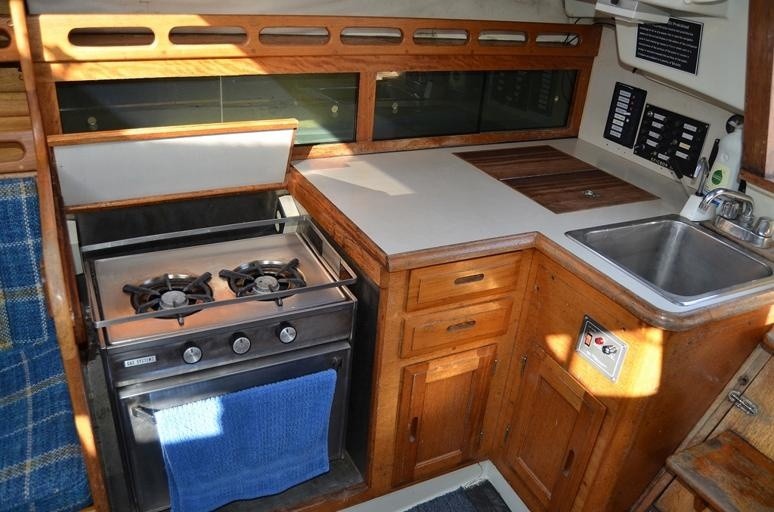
[{"x1": 119, "y1": 339, "x2": 353, "y2": 512}]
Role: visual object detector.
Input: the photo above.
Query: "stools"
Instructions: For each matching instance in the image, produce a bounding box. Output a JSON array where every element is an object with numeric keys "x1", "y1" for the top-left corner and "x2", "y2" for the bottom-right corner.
[{"x1": 633, "y1": 430, "x2": 774, "y2": 511}]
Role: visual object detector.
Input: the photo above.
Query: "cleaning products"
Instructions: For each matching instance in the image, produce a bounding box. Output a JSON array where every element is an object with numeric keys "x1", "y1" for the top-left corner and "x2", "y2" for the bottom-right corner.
[{"x1": 705, "y1": 113, "x2": 745, "y2": 201}]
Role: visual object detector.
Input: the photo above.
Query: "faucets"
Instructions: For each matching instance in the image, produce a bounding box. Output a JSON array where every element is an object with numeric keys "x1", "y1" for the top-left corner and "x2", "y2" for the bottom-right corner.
[
  {"x1": 693, "y1": 155, "x2": 710, "y2": 181},
  {"x1": 700, "y1": 188, "x2": 755, "y2": 224}
]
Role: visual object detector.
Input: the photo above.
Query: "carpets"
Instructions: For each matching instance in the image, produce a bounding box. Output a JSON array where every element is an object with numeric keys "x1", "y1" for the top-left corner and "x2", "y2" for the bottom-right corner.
[{"x1": 404, "y1": 481, "x2": 511, "y2": 512}]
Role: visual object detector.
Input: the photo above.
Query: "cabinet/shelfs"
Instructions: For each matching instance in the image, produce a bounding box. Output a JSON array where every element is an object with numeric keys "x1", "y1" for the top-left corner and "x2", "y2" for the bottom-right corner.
[
  {"x1": 629, "y1": 322, "x2": 773, "y2": 511},
  {"x1": 286, "y1": 161, "x2": 533, "y2": 495},
  {"x1": 494, "y1": 244, "x2": 772, "y2": 509}
]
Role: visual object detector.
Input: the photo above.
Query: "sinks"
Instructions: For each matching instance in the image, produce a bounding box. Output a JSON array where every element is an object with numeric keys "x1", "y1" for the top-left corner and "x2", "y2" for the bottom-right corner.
[{"x1": 566, "y1": 214, "x2": 774, "y2": 312}]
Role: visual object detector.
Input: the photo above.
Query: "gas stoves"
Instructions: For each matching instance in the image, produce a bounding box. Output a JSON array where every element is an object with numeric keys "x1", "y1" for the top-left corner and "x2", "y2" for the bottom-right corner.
[{"x1": 89, "y1": 232, "x2": 357, "y2": 388}]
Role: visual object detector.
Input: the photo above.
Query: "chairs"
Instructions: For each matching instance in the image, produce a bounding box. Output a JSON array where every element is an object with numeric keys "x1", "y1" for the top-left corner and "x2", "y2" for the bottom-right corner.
[{"x1": 0, "y1": 0, "x2": 111, "y2": 511}]
